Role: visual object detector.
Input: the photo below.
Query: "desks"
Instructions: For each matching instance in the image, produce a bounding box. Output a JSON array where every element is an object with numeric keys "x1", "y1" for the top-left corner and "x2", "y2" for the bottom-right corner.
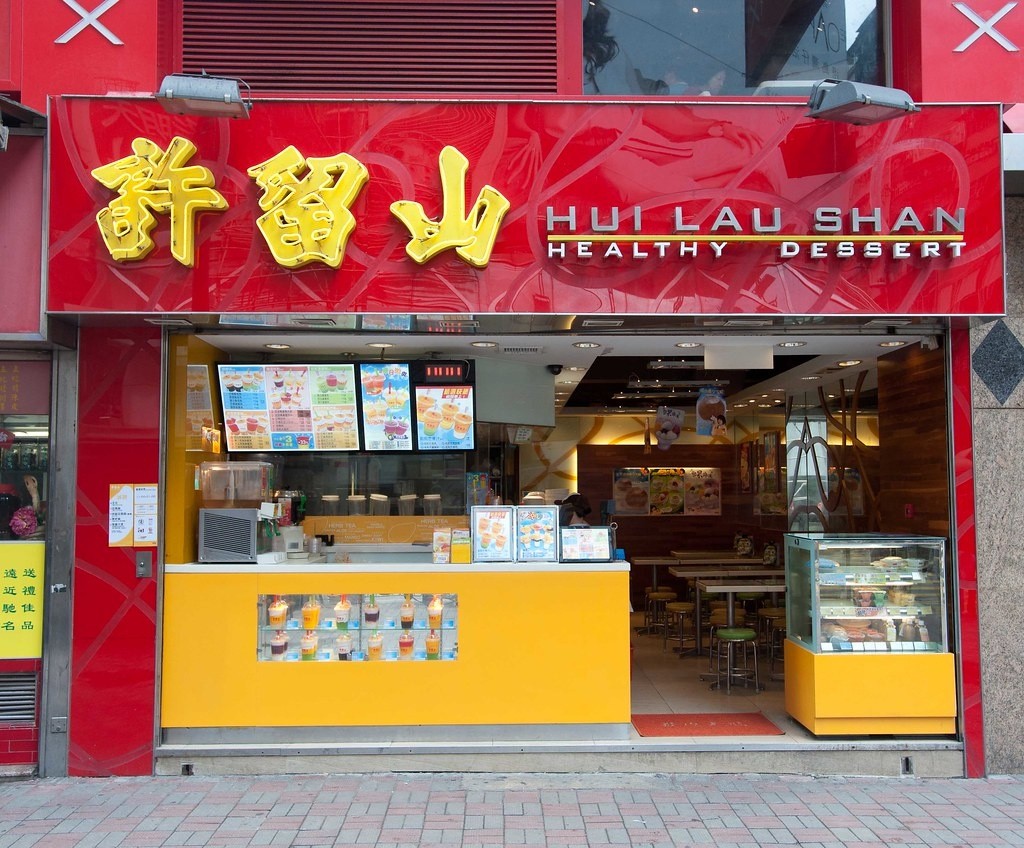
[{"x1": 630, "y1": 550, "x2": 786, "y2": 691}]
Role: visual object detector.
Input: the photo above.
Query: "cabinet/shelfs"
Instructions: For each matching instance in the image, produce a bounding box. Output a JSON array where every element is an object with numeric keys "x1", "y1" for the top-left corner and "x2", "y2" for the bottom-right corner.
[{"x1": 782, "y1": 531, "x2": 958, "y2": 736}]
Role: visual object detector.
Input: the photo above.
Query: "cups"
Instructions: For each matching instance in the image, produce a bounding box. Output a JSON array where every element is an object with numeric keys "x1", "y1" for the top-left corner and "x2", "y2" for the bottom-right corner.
[
  {"x1": 417, "y1": 396, "x2": 472, "y2": 440},
  {"x1": 334, "y1": 602, "x2": 350, "y2": 629},
  {"x1": 425, "y1": 635, "x2": 440, "y2": 659},
  {"x1": 428, "y1": 600, "x2": 443, "y2": 629},
  {"x1": 316, "y1": 373, "x2": 348, "y2": 393},
  {"x1": 270, "y1": 375, "x2": 305, "y2": 409},
  {"x1": 365, "y1": 603, "x2": 379, "y2": 626},
  {"x1": 227, "y1": 417, "x2": 269, "y2": 434},
  {"x1": 313, "y1": 414, "x2": 354, "y2": 431},
  {"x1": 400, "y1": 635, "x2": 414, "y2": 657},
  {"x1": 270, "y1": 631, "x2": 288, "y2": 660},
  {"x1": 224, "y1": 374, "x2": 263, "y2": 392},
  {"x1": 364, "y1": 374, "x2": 410, "y2": 436},
  {"x1": 301, "y1": 631, "x2": 317, "y2": 660},
  {"x1": 399, "y1": 604, "x2": 414, "y2": 629},
  {"x1": 301, "y1": 601, "x2": 320, "y2": 629},
  {"x1": 337, "y1": 635, "x2": 352, "y2": 660},
  {"x1": 268, "y1": 601, "x2": 288, "y2": 628},
  {"x1": 367, "y1": 634, "x2": 382, "y2": 659}
]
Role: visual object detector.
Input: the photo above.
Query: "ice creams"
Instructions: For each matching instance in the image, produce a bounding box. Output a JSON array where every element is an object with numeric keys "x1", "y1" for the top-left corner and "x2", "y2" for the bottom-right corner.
[{"x1": 656, "y1": 421, "x2": 677, "y2": 450}]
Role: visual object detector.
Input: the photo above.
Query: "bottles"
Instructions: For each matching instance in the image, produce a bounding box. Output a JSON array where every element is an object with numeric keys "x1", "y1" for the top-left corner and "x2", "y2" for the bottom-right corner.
[{"x1": 899, "y1": 618, "x2": 929, "y2": 641}]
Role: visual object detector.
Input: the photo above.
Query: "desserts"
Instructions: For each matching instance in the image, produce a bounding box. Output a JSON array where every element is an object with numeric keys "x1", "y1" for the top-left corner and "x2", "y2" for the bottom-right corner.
[{"x1": 821, "y1": 620, "x2": 884, "y2": 643}]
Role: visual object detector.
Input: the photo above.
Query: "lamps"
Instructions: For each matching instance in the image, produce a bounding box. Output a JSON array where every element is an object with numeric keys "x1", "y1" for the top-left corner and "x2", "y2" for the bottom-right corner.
[
  {"x1": 153, "y1": 68, "x2": 255, "y2": 119},
  {"x1": 803, "y1": 78, "x2": 921, "y2": 126}
]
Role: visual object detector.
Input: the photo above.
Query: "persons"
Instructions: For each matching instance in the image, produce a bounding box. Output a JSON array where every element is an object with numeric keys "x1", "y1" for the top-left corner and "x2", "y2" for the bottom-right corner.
[
  {"x1": 553, "y1": 491, "x2": 590, "y2": 526},
  {"x1": 710, "y1": 415, "x2": 726, "y2": 436}
]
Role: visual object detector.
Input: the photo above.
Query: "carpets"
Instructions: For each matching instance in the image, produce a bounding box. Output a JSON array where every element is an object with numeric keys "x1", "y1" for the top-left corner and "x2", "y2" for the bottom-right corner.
[{"x1": 631, "y1": 709, "x2": 787, "y2": 737}]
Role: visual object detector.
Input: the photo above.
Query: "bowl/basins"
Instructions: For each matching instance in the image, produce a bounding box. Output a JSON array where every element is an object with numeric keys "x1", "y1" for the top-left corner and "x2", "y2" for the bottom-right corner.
[{"x1": 836, "y1": 619, "x2": 871, "y2": 628}]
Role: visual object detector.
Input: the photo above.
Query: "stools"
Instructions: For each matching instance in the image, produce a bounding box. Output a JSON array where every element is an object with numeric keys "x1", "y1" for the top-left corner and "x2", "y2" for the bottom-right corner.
[{"x1": 643, "y1": 576, "x2": 786, "y2": 696}]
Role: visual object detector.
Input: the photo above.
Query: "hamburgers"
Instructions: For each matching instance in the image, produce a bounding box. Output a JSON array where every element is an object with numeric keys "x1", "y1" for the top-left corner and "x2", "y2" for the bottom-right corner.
[{"x1": 617, "y1": 479, "x2": 649, "y2": 510}]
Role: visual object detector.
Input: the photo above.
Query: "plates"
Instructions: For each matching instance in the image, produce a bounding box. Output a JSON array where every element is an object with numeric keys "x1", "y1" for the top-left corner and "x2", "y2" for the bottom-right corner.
[
  {"x1": 819, "y1": 560, "x2": 840, "y2": 570},
  {"x1": 545, "y1": 488, "x2": 568, "y2": 505}
]
